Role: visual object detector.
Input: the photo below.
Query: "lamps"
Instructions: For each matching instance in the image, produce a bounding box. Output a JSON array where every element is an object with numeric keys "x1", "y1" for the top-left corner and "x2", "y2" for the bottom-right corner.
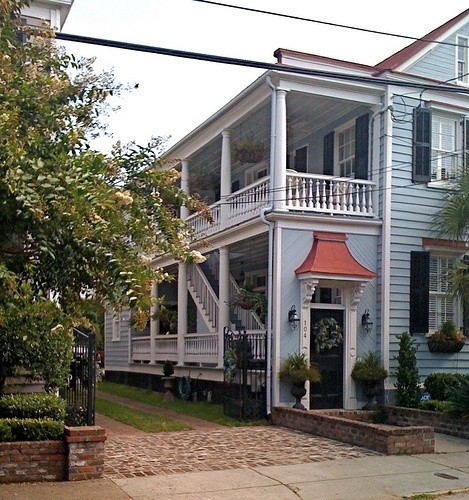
[
  {"x1": 288, "y1": 304, "x2": 298, "y2": 330},
  {"x1": 360, "y1": 310, "x2": 374, "y2": 333}
]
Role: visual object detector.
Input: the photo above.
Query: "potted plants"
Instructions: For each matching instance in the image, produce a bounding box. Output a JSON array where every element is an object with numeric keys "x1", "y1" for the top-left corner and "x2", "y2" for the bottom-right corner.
[
  {"x1": 231, "y1": 285, "x2": 260, "y2": 311},
  {"x1": 283, "y1": 353, "x2": 319, "y2": 408},
  {"x1": 161, "y1": 364, "x2": 176, "y2": 389},
  {"x1": 353, "y1": 354, "x2": 385, "y2": 410},
  {"x1": 427, "y1": 319, "x2": 466, "y2": 353}
]
